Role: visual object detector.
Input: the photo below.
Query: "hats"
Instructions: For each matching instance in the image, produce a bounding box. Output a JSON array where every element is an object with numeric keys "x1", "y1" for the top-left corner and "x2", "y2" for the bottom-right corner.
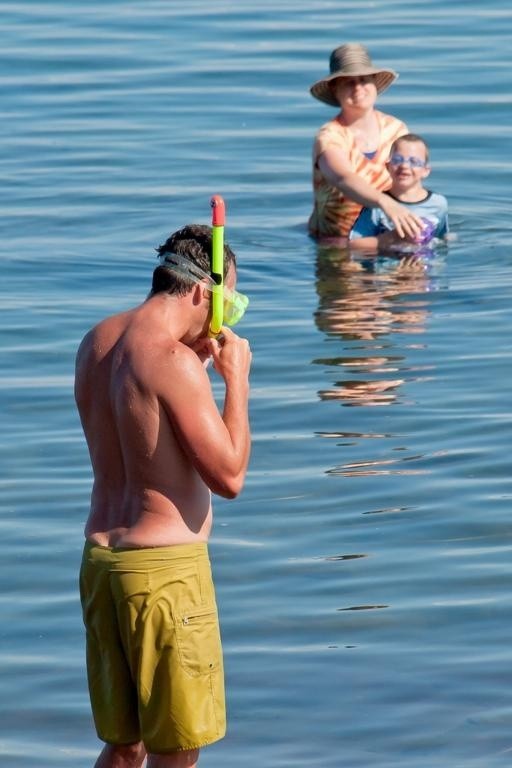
[{"x1": 310, "y1": 43, "x2": 397, "y2": 106}]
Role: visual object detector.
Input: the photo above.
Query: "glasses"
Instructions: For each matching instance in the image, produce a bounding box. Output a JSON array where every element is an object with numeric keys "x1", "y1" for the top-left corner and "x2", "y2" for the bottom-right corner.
[
  {"x1": 390, "y1": 156, "x2": 426, "y2": 169},
  {"x1": 158, "y1": 252, "x2": 249, "y2": 326}
]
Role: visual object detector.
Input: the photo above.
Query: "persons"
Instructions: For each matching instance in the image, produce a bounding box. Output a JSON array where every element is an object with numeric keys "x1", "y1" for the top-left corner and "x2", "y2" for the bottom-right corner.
[
  {"x1": 73, "y1": 225, "x2": 254, "y2": 768},
  {"x1": 305, "y1": 42, "x2": 427, "y2": 239},
  {"x1": 348, "y1": 133, "x2": 451, "y2": 251},
  {"x1": 311, "y1": 248, "x2": 428, "y2": 409}
]
